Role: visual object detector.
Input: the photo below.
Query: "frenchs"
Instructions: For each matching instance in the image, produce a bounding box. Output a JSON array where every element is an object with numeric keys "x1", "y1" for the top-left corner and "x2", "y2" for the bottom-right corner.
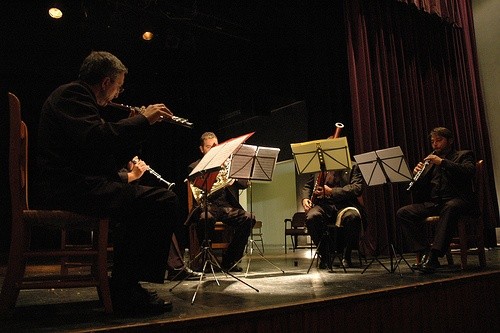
[{"x1": 190, "y1": 158, "x2": 236, "y2": 205}]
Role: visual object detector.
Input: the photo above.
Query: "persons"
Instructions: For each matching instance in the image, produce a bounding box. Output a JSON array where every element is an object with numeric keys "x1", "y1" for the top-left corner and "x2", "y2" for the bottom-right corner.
[
  {"x1": 299, "y1": 135, "x2": 366, "y2": 270},
  {"x1": 186, "y1": 131, "x2": 256, "y2": 274},
  {"x1": 394, "y1": 123, "x2": 478, "y2": 273},
  {"x1": 27, "y1": 50, "x2": 207, "y2": 318}
]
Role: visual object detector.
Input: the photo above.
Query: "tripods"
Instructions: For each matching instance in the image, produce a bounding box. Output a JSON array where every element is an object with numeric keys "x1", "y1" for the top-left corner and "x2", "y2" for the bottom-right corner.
[
  {"x1": 356, "y1": 151, "x2": 415, "y2": 278},
  {"x1": 169, "y1": 132, "x2": 285, "y2": 306},
  {"x1": 292, "y1": 146, "x2": 350, "y2": 274}
]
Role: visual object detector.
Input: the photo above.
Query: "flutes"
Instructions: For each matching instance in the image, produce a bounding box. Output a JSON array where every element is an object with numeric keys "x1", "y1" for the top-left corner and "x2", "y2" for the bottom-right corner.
[
  {"x1": 108, "y1": 102, "x2": 194, "y2": 128},
  {"x1": 406, "y1": 149, "x2": 437, "y2": 191}
]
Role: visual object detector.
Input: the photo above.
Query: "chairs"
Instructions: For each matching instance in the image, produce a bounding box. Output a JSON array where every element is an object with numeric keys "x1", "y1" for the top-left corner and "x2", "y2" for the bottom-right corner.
[
  {"x1": 284, "y1": 212, "x2": 313, "y2": 254},
  {"x1": 250, "y1": 220, "x2": 264, "y2": 254},
  {"x1": 353, "y1": 184, "x2": 367, "y2": 266},
  {"x1": 424, "y1": 159, "x2": 488, "y2": 269},
  {"x1": 187, "y1": 178, "x2": 232, "y2": 260},
  {"x1": 0, "y1": 92, "x2": 114, "y2": 315}
]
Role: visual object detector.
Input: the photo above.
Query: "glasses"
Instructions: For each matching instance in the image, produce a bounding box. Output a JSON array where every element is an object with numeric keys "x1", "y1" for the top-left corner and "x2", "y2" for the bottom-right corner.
[{"x1": 112, "y1": 79, "x2": 125, "y2": 93}]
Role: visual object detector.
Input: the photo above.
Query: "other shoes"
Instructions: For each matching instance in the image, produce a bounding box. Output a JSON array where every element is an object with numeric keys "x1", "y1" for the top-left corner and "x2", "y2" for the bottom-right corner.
[
  {"x1": 168, "y1": 265, "x2": 205, "y2": 282},
  {"x1": 221, "y1": 262, "x2": 244, "y2": 273},
  {"x1": 341, "y1": 255, "x2": 354, "y2": 268},
  {"x1": 319, "y1": 255, "x2": 328, "y2": 269},
  {"x1": 114, "y1": 292, "x2": 173, "y2": 316}
]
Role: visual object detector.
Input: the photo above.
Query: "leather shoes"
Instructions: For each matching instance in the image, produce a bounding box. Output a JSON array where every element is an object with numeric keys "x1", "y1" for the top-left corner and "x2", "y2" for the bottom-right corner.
[
  {"x1": 419, "y1": 256, "x2": 440, "y2": 271},
  {"x1": 411, "y1": 253, "x2": 427, "y2": 269}
]
